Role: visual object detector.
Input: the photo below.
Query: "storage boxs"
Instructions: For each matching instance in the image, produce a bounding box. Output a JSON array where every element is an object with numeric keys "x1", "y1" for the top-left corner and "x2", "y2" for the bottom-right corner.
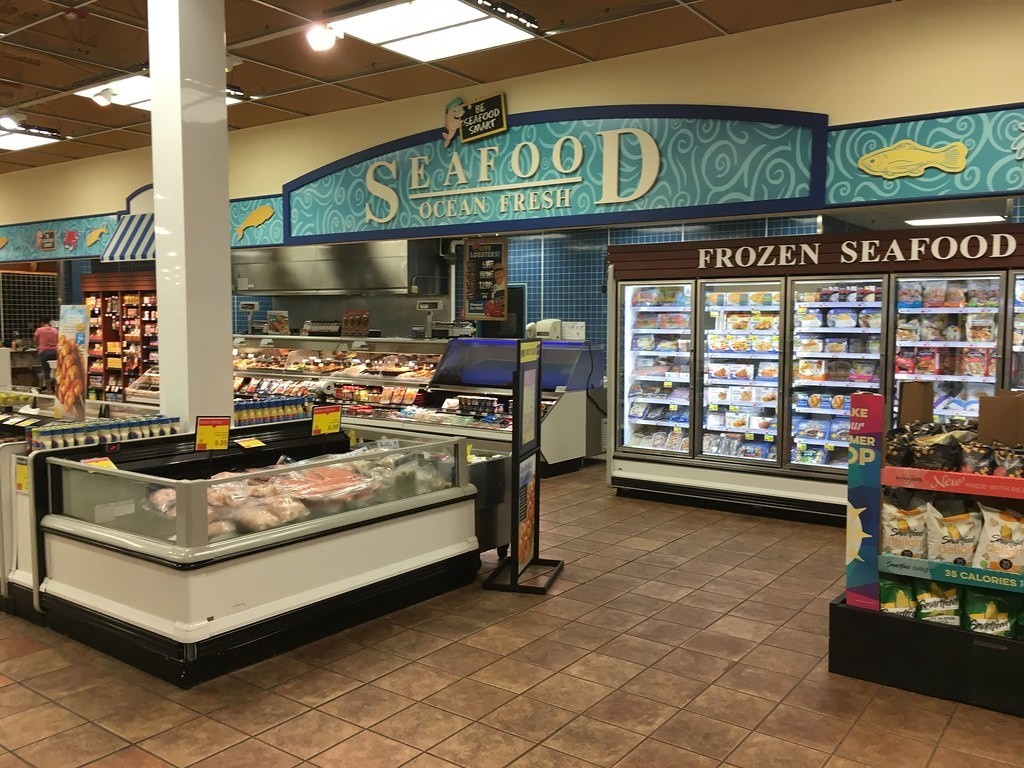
[{"x1": 845, "y1": 380, "x2": 1024, "y2": 643}]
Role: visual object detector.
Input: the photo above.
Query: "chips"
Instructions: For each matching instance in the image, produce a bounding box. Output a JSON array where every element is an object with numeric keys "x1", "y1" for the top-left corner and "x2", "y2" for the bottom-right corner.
[
  {"x1": 969, "y1": 601, "x2": 1009, "y2": 620},
  {"x1": 916, "y1": 582, "x2": 956, "y2": 600},
  {"x1": 880, "y1": 590, "x2": 917, "y2": 609}
]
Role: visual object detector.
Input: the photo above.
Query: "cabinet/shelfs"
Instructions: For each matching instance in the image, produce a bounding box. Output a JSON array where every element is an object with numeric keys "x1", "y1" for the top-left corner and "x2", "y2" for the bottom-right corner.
[
  {"x1": 624, "y1": 307, "x2": 691, "y2": 450},
  {"x1": 702, "y1": 307, "x2": 780, "y2": 458},
  {"x1": 80, "y1": 273, "x2": 160, "y2": 403},
  {"x1": 893, "y1": 306, "x2": 998, "y2": 418},
  {"x1": 791, "y1": 301, "x2": 881, "y2": 465},
  {"x1": 1010, "y1": 306, "x2": 1024, "y2": 391}
]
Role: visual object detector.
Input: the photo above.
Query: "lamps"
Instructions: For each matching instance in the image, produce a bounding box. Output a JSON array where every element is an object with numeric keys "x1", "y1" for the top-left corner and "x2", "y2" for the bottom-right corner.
[{"x1": 93, "y1": 89, "x2": 114, "y2": 106}]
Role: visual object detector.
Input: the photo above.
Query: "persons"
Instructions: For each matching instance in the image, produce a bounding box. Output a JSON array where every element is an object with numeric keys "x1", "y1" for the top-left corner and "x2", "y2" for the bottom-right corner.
[{"x1": 33, "y1": 317, "x2": 58, "y2": 394}]
